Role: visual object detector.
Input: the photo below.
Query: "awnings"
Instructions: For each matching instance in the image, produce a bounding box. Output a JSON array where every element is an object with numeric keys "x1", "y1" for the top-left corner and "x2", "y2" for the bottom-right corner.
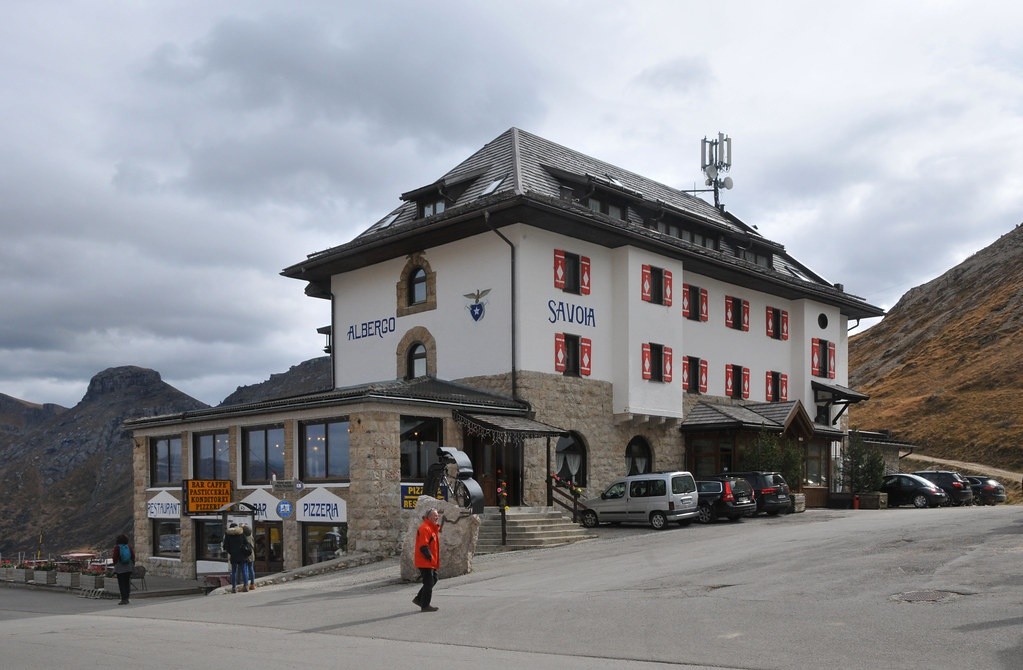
[{"x1": 810, "y1": 379, "x2": 868, "y2": 425}]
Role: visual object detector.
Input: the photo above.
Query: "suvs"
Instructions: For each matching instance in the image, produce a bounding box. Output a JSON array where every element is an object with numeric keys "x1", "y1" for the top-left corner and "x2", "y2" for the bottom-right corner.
[
  {"x1": 694, "y1": 474, "x2": 758, "y2": 524},
  {"x1": 908, "y1": 470, "x2": 973, "y2": 507},
  {"x1": 716, "y1": 471, "x2": 792, "y2": 516},
  {"x1": 577, "y1": 469, "x2": 701, "y2": 530},
  {"x1": 963, "y1": 474, "x2": 1006, "y2": 506}
]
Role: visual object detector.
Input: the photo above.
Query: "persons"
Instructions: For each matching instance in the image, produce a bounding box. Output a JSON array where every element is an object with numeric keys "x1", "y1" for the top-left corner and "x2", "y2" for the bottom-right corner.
[
  {"x1": 223, "y1": 523, "x2": 256, "y2": 594},
  {"x1": 113, "y1": 534, "x2": 135, "y2": 605},
  {"x1": 412, "y1": 508, "x2": 441, "y2": 612}
]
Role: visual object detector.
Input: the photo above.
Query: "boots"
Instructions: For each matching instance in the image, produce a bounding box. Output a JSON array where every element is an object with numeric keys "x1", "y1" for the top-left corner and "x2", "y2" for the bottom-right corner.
[
  {"x1": 238, "y1": 585, "x2": 249, "y2": 592},
  {"x1": 249, "y1": 583, "x2": 256, "y2": 590}
]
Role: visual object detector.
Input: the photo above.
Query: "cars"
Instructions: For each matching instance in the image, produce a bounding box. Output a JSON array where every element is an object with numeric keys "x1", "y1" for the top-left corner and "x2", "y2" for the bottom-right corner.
[{"x1": 858, "y1": 472, "x2": 950, "y2": 509}]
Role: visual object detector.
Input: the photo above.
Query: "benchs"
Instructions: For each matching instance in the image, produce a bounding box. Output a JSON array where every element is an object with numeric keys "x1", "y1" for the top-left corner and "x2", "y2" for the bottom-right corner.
[{"x1": 199, "y1": 586, "x2": 218, "y2": 595}]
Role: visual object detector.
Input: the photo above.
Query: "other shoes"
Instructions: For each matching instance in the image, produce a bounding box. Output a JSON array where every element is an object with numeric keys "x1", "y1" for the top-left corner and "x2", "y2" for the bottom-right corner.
[
  {"x1": 413, "y1": 597, "x2": 421, "y2": 608},
  {"x1": 231, "y1": 587, "x2": 237, "y2": 593},
  {"x1": 421, "y1": 605, "x2": 438, "y2": 612},
  {"x1": 118, "y1": 601, "x2": 129, "y2": 604}
]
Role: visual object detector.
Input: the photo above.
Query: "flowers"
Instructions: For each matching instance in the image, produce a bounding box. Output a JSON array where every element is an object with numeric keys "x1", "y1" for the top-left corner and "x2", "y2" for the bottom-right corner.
[
  {"x1": 81, "y1": 564, "x2": 104, "y2": 576},
  {"x1": 550, "y1": 471, "x2": 583, "y2": 494},
  {"x1": 106, "y1": 569, "x2": 118, "y2": 578},
  {"x1": 16, "y1": 559, "x2": 32, "y2": 569},
  {"x1": 0, "y1": 560, "x2": 14, "y2": 568},
  {"x1": 57, "y1": 564, "x2": 79, "y2": 572},
  {"x1": 496, "y1": 476, "x2": 509, "y2": 512}
]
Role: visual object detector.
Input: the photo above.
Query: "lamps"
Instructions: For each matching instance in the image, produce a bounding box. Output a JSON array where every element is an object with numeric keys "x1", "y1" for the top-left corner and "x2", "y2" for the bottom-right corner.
[{"x1": 120, "y1": 429, "x2": 140, "y2": 447}]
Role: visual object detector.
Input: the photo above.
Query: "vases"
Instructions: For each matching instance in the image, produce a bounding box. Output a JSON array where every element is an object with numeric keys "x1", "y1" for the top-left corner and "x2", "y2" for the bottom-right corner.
[
  {"x1": 104, "y1": 577, "x2": 145, "y2": 592},
  {"x1": 15, "y1": 569, "x2": 33, "y2": 582},
  {"x1": 0, "y1": 569, "x2": 16, "y2": 580},
  {"x1": 80, "y1": 574, "x2": 105, "y2": 590},
  {"x1": 34, "y1": 570, "x2": 56, "y2": 584},
  {"x1": 57, "y1": 572, "x2": 80, "y2": 586}
]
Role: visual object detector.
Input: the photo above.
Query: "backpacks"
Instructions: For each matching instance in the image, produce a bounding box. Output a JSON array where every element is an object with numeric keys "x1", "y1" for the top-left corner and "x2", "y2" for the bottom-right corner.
[{"x1": 118, "y1": 544, "x2": 131, "y2": 564}]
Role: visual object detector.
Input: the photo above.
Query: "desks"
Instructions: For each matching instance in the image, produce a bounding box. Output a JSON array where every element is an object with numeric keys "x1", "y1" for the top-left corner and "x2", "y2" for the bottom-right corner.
[{"x1": 206, "y1": 575, "x2": 230, "y2": 586}]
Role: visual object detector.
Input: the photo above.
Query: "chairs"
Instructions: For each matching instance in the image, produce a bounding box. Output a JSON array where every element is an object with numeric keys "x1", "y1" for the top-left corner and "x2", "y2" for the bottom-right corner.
[{"x1": 130, "y1": 566, "x2": 147, "y2": 591}]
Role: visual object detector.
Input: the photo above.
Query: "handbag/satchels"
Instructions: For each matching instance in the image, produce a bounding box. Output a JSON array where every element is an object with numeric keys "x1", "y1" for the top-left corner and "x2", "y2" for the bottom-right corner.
[{"x1": 243, "y1": 543, "x2": 252, "y2": 556}]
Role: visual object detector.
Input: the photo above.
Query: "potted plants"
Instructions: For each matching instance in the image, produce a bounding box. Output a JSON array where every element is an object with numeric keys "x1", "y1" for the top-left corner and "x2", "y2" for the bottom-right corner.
[
  {"x1": 742, "y1": 420, "x2": 808, "y2": 512},
  {"x1": 827, "y1": 424, "x2": 890, "y2": 510},
  {"x1": 33, "y1": 562, "x2": 56, "y2": 584}
]
